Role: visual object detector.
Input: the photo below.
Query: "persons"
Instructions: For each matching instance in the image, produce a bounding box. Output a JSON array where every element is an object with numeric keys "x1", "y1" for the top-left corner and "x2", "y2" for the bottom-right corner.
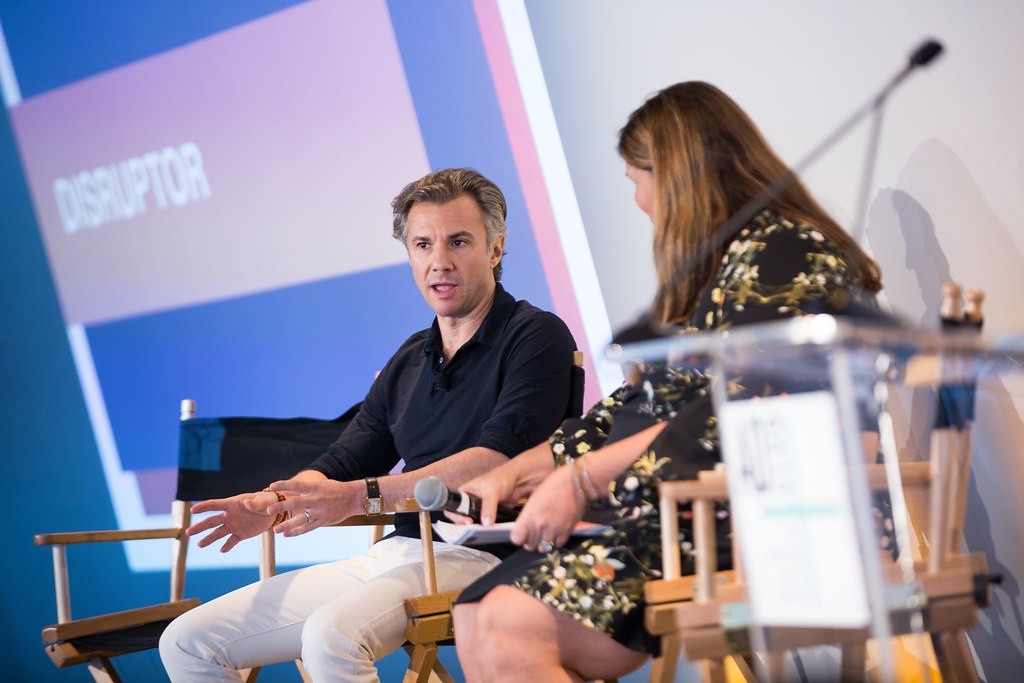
[
  {"x1": 159, "y1": 169, "x2": 583, "y2": 683},
  {"x1": 452, "y1": 81, "x2": 887, "y2": 682}
]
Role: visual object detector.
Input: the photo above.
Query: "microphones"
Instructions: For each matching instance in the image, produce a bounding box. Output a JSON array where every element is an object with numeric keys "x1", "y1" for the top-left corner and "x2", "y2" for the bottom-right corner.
[
  {"x1": 434, "y1": 372, "x2": 451, "y2": 389},
  {"x1": 415, "y1": 478, "x2": 521, "y2": 526},
  {"x1": 608, "y1": 42, "x2": 944, "y2": 346}
]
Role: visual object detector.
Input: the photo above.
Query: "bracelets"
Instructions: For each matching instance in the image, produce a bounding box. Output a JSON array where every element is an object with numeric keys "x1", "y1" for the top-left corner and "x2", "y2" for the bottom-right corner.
[
  {"x1": 571, "y1": 455, "x2": 600, "y2": 505},
  {"x1": 263, "y1": 488, "x2": 286, "y2": 527}
]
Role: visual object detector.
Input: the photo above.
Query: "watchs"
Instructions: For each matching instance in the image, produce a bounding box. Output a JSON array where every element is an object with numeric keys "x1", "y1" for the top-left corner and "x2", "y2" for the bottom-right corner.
[{"x1": 365, "y1": 477, "x2": 383, "y2": 517}]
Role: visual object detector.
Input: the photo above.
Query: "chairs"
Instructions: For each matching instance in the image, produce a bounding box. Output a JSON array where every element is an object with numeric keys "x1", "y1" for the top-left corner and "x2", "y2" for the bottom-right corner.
[{"x1": 36, "y1": 287, "x2": 992, "y2": 683}]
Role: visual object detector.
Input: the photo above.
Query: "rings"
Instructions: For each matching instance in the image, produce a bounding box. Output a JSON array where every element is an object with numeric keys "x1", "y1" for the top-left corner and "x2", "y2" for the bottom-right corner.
[
  {"x1": 305, "y1": 512, "x2": 312, "y2": 523},
  {"x1": 540, "y1": 540, "x2": 554, "y2": 552}
]
